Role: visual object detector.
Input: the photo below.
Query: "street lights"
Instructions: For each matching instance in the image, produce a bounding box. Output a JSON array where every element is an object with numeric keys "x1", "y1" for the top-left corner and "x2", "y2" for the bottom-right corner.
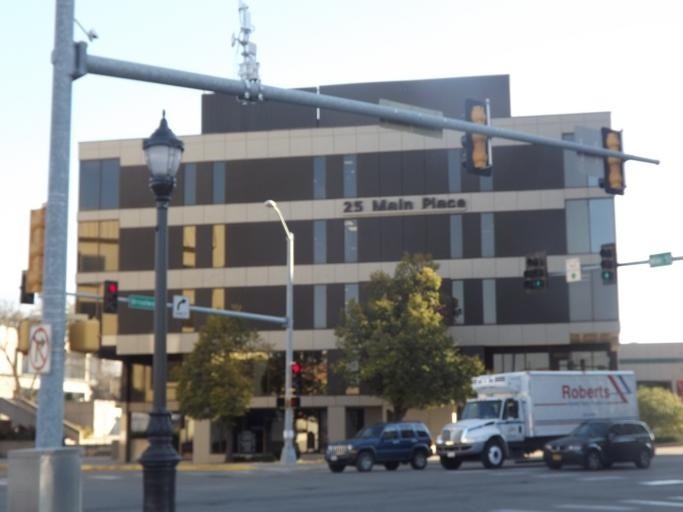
[
  {"x1": 261, "y1": 195, "x2": 295, "y2": 464},
  {"x1": 138, "y1": 109, "x2": 186, "y2": 512}
]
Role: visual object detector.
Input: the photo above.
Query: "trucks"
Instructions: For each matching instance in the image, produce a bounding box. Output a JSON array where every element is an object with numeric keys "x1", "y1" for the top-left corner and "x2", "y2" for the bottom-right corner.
[{"x1": 431, "y1": 367, "x2": 642, "y2": 469}]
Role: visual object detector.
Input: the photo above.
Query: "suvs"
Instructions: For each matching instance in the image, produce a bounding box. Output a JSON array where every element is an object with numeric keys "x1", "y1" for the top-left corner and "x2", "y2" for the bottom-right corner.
[
  {"x1": 323, "y1": 421, "x2": 434, "y2": 471},
  {"x1": 540, "y1": 419, "x2": 655, "y2": 469}
]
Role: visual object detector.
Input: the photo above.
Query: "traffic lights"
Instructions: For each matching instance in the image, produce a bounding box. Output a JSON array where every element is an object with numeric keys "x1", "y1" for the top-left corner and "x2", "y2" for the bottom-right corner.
[
  {"x1": 458, "y1": 98, "x2": 490, "y2": 174},
  {"x1": 292, "y1": 363, "x2": 299, "y2": 388},
  {"x1": 595, "y1": 126, "x2": 625, "y2": 194},
  {"x1": 103, "y1": 281, "x2": 115, "y2": 313},
  {"x1": 600, "y1": 247, "x2": 614, "y2": 281},
  {"x1": 522, "y1": 257, "x2": 544, "y2": 288},
  {"x1": 19, "y1": 269, "x2": 33, "y2": 304}
]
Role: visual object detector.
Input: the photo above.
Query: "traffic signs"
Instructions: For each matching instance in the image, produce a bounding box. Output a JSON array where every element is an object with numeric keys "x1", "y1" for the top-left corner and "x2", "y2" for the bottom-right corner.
[
  {"x1": 648, "y1": 253, "x2": 671, "y2": 269},
  {"x1": 127, "y1": 295, "x2": 156, "y2": 310}
]
